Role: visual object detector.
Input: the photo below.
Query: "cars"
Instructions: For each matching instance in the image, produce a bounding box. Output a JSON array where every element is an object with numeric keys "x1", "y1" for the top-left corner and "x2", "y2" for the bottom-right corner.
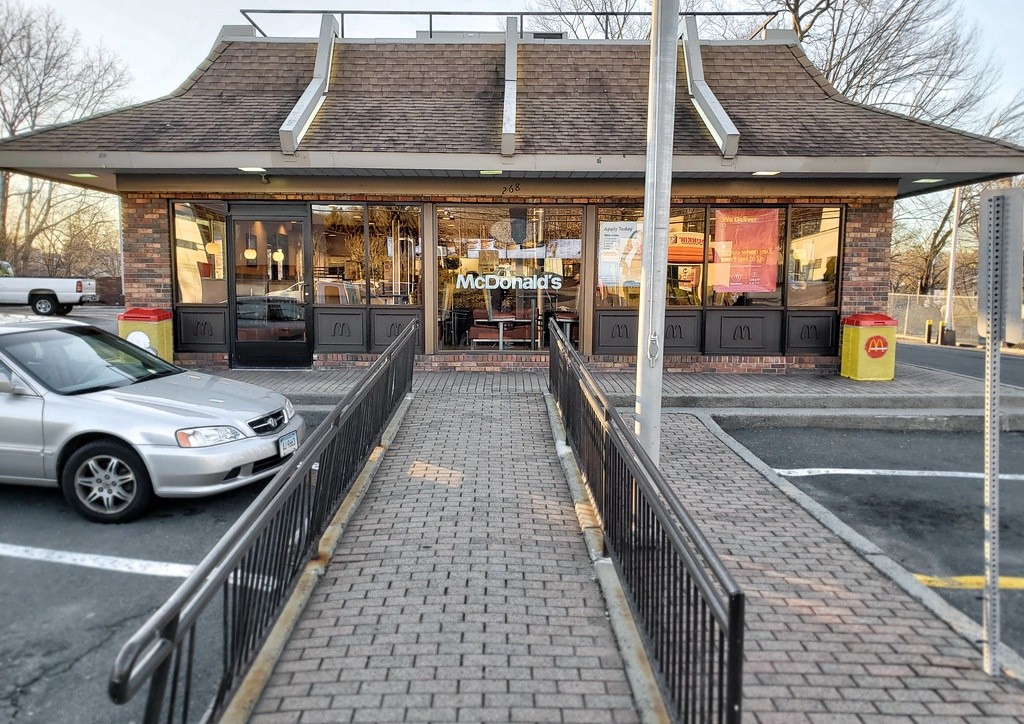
[
  {"x1": 220, "y1": 273, "x2": 393, "y2": 341},
  {"x1": 0, "y1": 315, "x2": 307, "y2": 523}
]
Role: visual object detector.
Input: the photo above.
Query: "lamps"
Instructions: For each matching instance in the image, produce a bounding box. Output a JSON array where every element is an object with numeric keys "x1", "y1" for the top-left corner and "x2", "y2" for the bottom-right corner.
[
  {"x1": 205, "y1": 218, "x2": 221, "y2": 255},
  {"x1": 273, "y1": 232, "x2": 285, "y2": 262},
  {"x1": 243, "y1": 221, "x2": 258, "y2": 260}
]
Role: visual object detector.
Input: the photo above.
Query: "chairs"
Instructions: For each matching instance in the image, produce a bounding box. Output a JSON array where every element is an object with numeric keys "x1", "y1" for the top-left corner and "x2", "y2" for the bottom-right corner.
[{"x1": 437, "y1": 274, "x2": 579, "y2": 351}]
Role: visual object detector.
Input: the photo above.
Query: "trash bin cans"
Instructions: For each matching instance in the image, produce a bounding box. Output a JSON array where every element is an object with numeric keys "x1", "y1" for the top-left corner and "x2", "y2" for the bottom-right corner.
[
  {"x1": 840, "y1": 313, "x2": 898, "y2": 381},
  {"x1": 117, "y1": 307, "x2": 173, "y2": 366}
]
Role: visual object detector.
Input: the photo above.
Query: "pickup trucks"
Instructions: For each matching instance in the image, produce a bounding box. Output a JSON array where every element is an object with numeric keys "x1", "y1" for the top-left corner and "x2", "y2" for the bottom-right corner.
[{"x1": 0, "y1": 260, "x2": 97, "y2": 315}]
